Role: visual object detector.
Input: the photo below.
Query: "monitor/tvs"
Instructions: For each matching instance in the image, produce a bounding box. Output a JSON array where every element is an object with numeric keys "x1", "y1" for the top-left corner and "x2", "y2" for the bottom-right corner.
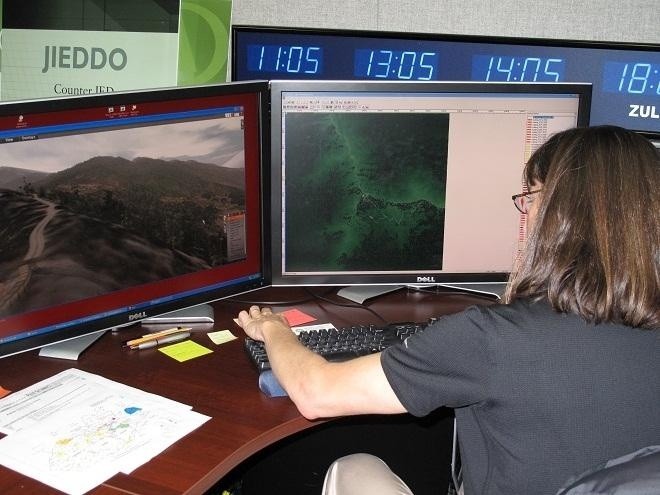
[
  {"x1": 270, "y1": 77, "x2": 594, "y2": 305},
  {"x1": 0, "y1": 77, "x2": 271, "y2": 361}
]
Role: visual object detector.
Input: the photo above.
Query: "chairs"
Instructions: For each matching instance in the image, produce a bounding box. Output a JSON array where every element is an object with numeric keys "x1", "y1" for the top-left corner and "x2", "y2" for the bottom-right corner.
[{"x1": 555, "y1": 446, "x2": 660, "y2": 495}]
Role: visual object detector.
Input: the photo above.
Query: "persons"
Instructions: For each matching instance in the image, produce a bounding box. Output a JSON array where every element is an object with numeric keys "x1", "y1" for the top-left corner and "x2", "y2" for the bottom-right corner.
[{"x1": 237, "y1": 124, "x2": 660, "y2": 494}]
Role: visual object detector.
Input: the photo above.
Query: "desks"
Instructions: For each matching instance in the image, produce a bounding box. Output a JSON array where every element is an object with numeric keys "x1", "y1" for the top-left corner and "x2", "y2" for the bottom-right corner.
[{"x1": 0, "y1": 284, "x2": 501, "y2": 495}]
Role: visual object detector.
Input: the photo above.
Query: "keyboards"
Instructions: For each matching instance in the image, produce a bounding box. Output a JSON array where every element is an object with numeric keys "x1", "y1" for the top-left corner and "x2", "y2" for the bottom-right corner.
[{"x1": 241, "y1": 316, "x2": 487, "y2": 374}]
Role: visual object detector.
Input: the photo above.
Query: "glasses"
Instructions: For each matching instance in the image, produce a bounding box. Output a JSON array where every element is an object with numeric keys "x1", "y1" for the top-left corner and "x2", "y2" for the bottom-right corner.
[{"x1": 512, "y1": 189, "x2": 540, "y2": 214}]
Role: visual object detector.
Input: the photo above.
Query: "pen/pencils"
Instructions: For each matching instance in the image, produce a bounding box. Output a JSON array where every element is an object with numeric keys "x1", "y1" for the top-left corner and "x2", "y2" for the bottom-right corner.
[{"x1": 120, "y1": 327, "x2": 192, "y2": 350}]
